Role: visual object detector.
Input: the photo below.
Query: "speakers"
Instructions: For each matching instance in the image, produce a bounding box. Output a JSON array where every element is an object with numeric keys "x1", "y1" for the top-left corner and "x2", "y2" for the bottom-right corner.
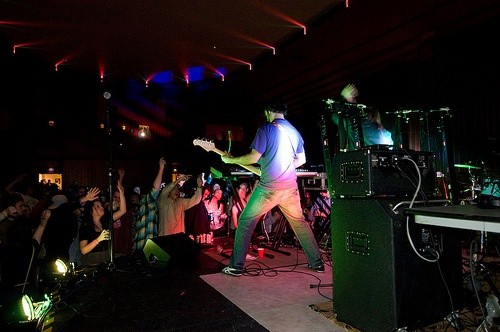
[
  {"x1": 331, "y1": 150, "x2": 437, "y2": 199},
  {"x1": 330, "y1": 197, "x2": 464, "y2": 332},
  {"x1": 143, "y1": 232, "x2": 200, "y2": 269}
]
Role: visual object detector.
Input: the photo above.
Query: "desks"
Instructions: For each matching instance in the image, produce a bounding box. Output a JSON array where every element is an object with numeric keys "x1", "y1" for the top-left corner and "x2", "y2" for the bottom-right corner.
[{"x1": 406, "y1": 204, "x2": 500, "y2": 238}]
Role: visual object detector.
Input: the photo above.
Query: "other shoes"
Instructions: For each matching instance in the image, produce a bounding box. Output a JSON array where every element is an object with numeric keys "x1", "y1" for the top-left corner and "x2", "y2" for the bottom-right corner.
[
  {"x1": 308, "y1": 264, "x2": 325, "y2": 272},
  {"x1": 222, "y1": 266, "x2": 243, "y2": 276}
]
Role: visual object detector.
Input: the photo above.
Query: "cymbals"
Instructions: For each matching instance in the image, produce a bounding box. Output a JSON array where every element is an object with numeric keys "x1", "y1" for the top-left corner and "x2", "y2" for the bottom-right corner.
[{"x1": 454, "y1": 164, "x2": 480, "y2": 169}]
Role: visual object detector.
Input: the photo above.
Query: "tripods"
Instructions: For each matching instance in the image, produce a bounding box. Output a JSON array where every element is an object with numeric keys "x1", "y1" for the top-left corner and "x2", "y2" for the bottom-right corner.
[{"x1": 55, "y1": 100, "x2": 157, "y2": 307}]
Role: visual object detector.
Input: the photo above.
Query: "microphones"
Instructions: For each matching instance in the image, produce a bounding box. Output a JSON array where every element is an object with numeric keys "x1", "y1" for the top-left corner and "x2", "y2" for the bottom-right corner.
[{"x1": 104, "y1": 92, "x2": 112, "y2": 99}]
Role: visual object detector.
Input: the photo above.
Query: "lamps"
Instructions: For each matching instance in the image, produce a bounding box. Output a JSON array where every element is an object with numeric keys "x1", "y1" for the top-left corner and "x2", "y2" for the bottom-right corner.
[{"x1": 21, "y1": 294, "x2": 47, "y2": 320}]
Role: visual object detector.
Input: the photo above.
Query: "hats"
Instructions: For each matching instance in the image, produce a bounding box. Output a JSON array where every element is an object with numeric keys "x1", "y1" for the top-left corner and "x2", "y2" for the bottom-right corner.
[{"x1": 48, "y1": 195, "x2": 68, "y2": 209}]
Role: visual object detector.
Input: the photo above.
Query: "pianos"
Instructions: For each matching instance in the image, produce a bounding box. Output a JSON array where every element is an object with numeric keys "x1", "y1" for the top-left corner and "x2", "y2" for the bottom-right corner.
[{"x1": 230, "y1": 169, "x2": 318, "y2": 177}]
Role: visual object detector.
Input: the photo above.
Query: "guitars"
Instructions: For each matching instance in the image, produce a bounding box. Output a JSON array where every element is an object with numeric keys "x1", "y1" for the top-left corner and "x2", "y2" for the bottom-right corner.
[{"x1": 192, "y1": 138, "x2": 262, "y2": 177}]
